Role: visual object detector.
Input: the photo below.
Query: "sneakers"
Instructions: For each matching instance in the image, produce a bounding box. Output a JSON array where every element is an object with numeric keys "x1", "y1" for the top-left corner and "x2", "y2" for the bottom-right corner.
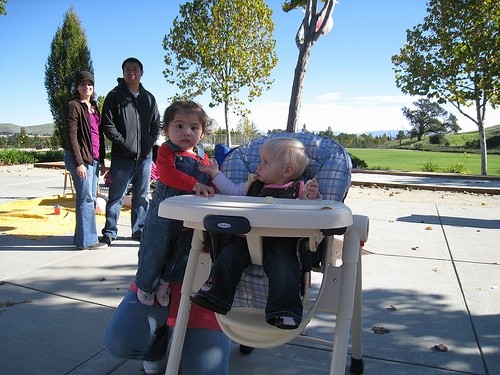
[{"x1": 142, "y1": 326, "x2": 175, "y2": 374}]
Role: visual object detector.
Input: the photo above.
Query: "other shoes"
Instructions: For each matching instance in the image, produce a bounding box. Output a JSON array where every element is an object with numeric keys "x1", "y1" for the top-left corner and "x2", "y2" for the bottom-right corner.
[
  {"x1": 136, "y1": 287, "x2": 155, "y2": 306},
  {"x1": 189, "y1": 291, "x2": 228, "y2": 315},
  {"x1": 77, "y1": 242, "x2": 108, "y2": 250},
  {"x1": 100, "y1": 234, "x2": 113, "y2": 246},
  {"x1": 156, "y1": 278, "x2": 171, "y2": 307},
  {"x1": 131, "y1": 231, "x2": 143, "y2": 240},
  {"x1": 267, "y1": 311, "x2": 302, "y2": 330}
]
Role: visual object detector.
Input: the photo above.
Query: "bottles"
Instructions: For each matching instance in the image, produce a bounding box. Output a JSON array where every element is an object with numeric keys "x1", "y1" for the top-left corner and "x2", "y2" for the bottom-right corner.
[{"x1": 55, "y1": 204, "x2": 60, "y2": 215}]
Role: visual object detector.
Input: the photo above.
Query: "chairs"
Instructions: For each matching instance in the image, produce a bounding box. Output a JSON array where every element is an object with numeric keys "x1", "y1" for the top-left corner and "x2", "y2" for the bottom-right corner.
[{"x1": 157, "y1": 132, "x2": 369, "y2": 375}]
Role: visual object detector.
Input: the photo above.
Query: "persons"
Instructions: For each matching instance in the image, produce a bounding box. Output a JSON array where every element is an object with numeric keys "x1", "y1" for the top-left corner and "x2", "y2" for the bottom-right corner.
[
  {"x1": 104, "y1": 249, "x2": 230, "y2": 375},
  {"x1": 98, "y1": 56, "x2": 160, "y2": 246},
  {"x1": 135, "y1": 100, "x2": 216, "y2": 307},
  {"x1": 196, "y1": 136, "x2": 321, "y2": 330},
  {"x1": 61, "y1": 71, "x2": 109, "y2": 250}
]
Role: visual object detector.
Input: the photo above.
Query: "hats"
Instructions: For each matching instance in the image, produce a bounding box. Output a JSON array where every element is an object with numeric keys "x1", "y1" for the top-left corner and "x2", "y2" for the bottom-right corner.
[{"x1": 75, "y1": 70, "x2": 95, "y2": 89}]
